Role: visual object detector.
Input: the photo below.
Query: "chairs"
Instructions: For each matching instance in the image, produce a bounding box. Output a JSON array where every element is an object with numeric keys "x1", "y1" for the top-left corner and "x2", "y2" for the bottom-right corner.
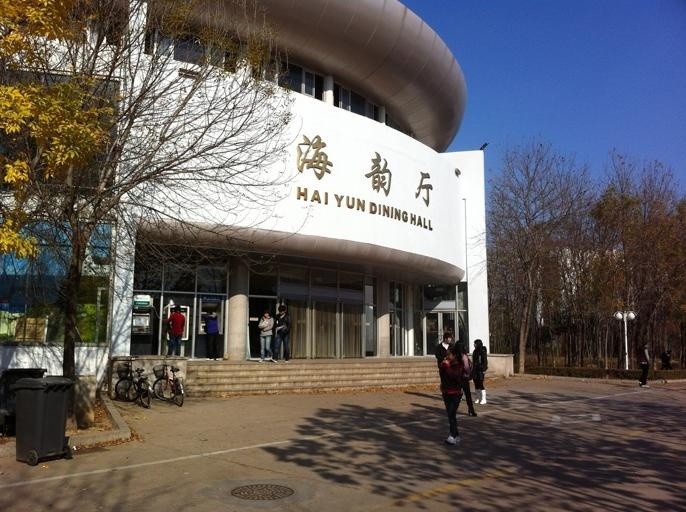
[
  {"x1": 638, "y1": 382, "x2": 650, "y2": 389},
  {"x1": 444, "y1": 434, "x2": 461, "y2": 445},
  {"x1": 258, "y1": 356, "x2": 291, "y2": 364},
  {"x1": 205, "y1": 357, "x2": 219, "y2": 362}
]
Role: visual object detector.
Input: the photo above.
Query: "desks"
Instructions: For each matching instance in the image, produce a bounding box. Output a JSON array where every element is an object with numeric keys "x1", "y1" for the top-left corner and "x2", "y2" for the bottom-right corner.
[{"x1": 613, "y1": 307, "x2": 640, "y2": 369}]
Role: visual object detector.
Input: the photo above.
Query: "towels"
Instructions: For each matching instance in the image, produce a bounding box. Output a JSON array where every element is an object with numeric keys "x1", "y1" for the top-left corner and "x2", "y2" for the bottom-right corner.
[{"x1": 0, "y1": 369, "x2": 76, "y2": 466}]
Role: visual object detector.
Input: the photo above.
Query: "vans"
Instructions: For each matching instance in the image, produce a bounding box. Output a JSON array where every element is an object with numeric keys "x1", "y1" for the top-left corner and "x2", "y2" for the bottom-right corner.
[{"x1": 468, "y1": 408, "x2": 477, "y2": 419}]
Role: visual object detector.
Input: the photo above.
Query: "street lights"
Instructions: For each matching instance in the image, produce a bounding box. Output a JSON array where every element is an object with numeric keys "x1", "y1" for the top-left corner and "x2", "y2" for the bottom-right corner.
[{"x1": 464, "y1": 368, "x2": 473, "y2": 381}]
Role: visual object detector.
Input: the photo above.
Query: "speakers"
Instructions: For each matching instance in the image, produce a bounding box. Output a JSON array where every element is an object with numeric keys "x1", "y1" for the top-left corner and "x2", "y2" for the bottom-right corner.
[{"x1": 474, "y1": 388, "x2": 487, "y2": 406}]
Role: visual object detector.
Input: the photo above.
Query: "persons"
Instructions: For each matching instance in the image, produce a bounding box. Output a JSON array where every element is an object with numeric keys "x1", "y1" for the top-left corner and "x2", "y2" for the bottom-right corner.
[
  {"x1": 205, "y1": 310, "x2": 219, "y2": 359},
  {"x1": 257, "y1": 310, "x2": 274, "y2": 363},
  {"x1": 163, "y1": 306, "x2": 185, "y2": 356},
  {"x1": 271, "y1": 305, "x2": 292, "y2": 364},
  {"x1": 637, "y1": 341, "x2": 651, "y2": 389},
  {"x1": 434, "y1": 332, "x2": 488, "y2": 445}
]
